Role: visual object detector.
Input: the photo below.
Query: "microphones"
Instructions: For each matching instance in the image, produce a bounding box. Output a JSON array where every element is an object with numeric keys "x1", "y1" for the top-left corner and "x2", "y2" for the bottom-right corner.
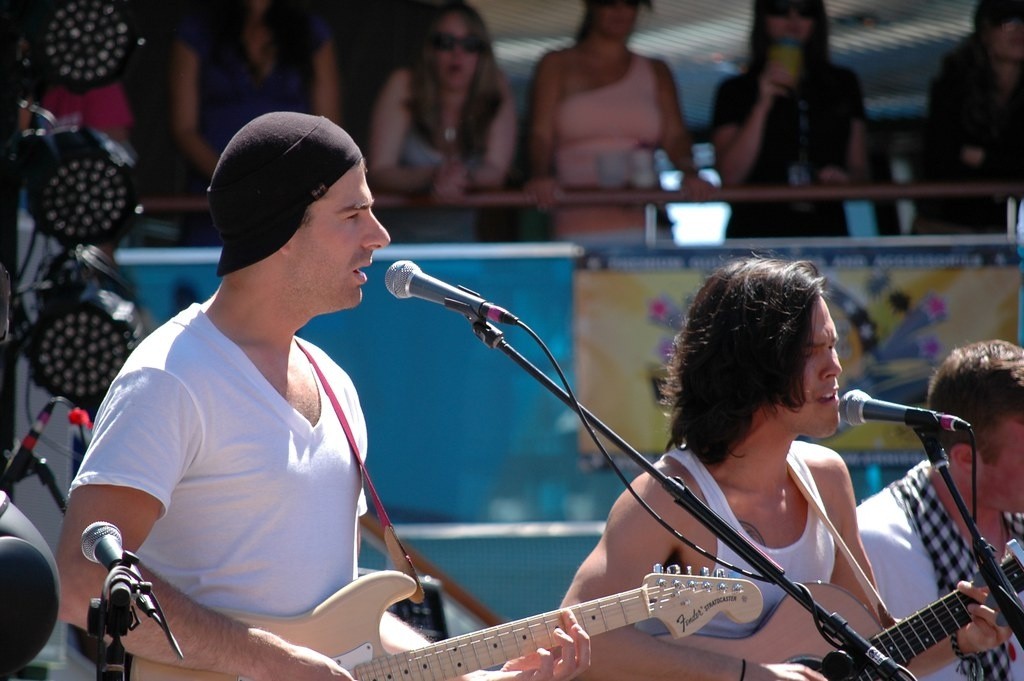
[
  {"x1": 838, "y1": 389, "x2": 971, "y2": 433},
  {"x1": 0, "y1": 399, "x2": 57, "y2": 496},
  {"x1": 385, "y1": 259, "x2": 519, "y2": 326},
  {"x1": 80, "y1": 520, "x2": 157, "y2": 618}
]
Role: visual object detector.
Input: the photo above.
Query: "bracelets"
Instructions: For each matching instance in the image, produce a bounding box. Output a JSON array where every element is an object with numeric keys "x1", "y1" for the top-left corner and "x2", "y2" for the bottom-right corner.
[
  {"x1": 740, "y1": 659, "x2": 746, "y2": 681},
  {"x1": 951, "y1": 632, "x2": 984, "y2": 680}
]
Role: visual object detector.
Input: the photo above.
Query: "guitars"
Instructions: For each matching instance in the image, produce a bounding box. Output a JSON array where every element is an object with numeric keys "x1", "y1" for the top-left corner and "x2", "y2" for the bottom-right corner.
[
  {"x1": 783, "y1": 538, "x2": 1018, "y2": 680},
  {"x1": 128, "y1": 561, "x2": 764, "y2": 680}
]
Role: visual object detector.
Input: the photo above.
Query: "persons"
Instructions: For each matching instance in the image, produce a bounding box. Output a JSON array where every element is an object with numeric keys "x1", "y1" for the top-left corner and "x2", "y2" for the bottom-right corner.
[
  {"x1": 15, "y1": 89, "x2": 135, "y2": 163},
  {"x1": 523, "y1": 1, "x2": 717, "y2": 241},
  {"x1": 57, "y1": 113, "x2": 593, "y2": 681},
  {"x1": 560, "y1": 260, "x2": 1012, "y2": 681},
  {"x1": 711, "y1": 0, "x2": 870, "y2": 239},
  {"x1": 169, "y1": 0, "x2": 514, "y2": 245},
  {"x1": 856, "y1": 340, "x2": 1024, "y2": 681},
  {"x1": 911, "y1": 0, "x2": 1024, "y2": 236}
]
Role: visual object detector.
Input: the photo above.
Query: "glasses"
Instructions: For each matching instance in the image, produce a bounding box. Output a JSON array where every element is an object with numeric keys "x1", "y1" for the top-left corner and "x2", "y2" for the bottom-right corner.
[{"x1": 426, "y1": 31, "x2": 484, "y2": 54}]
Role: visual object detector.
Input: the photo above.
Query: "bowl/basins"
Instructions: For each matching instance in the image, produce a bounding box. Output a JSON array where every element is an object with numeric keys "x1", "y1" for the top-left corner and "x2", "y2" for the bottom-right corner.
[{"x1": 665, "y1": 200, "x2": 732, "y2": 248}]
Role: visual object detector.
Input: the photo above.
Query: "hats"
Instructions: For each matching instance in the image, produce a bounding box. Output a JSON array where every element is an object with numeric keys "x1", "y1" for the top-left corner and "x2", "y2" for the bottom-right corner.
[{"x1": 205, "y1": 111, "x2": 364, "y2": 277}]
